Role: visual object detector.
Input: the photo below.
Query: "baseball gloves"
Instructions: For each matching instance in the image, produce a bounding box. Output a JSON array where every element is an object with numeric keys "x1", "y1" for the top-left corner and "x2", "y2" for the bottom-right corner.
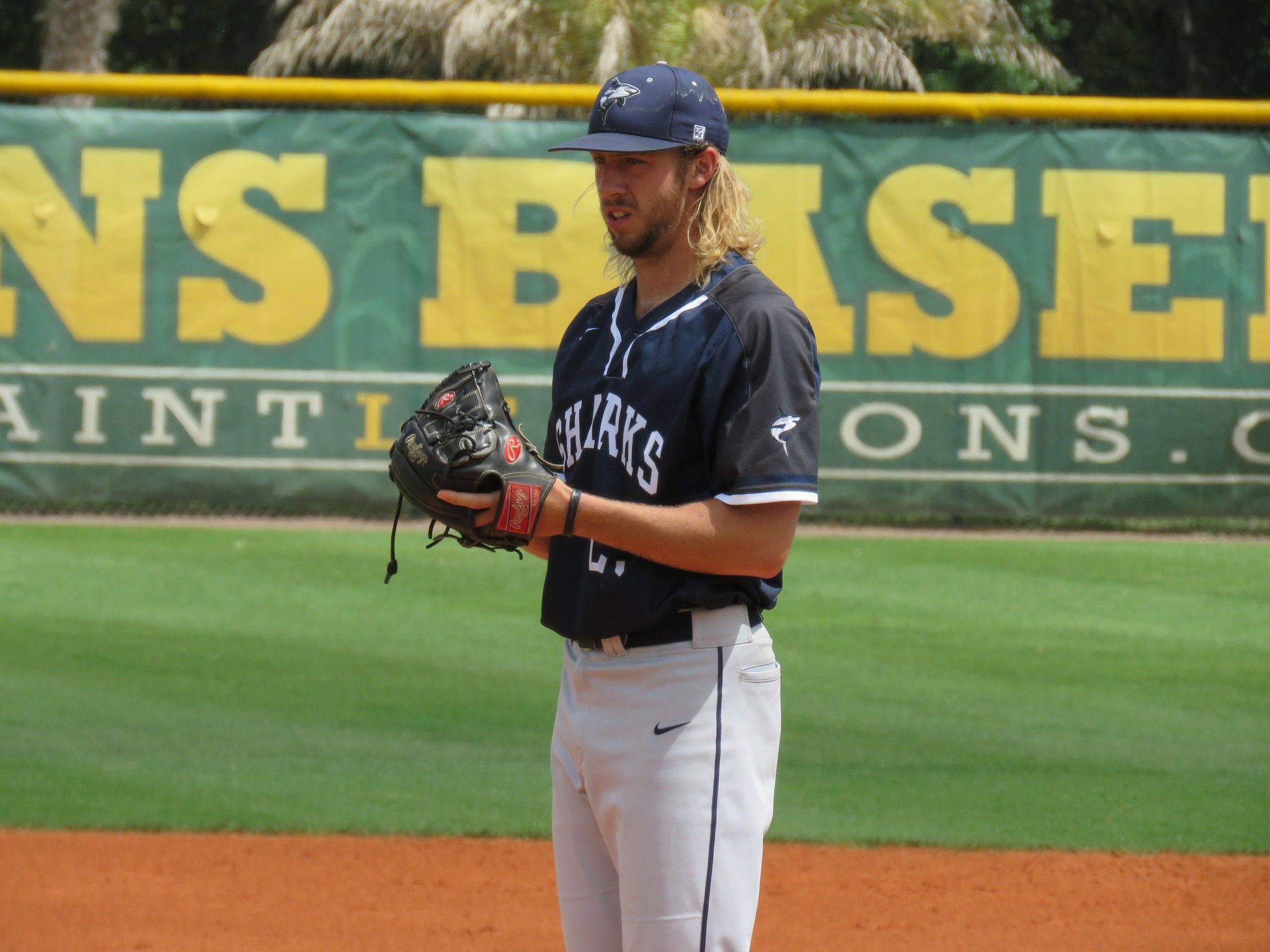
[{"x1": 384, "y1": 360, "x2": 566, "y2": 553}]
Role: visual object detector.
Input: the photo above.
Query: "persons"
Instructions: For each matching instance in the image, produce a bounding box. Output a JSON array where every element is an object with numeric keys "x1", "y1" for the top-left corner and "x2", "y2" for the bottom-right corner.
[{"x1": 385, "y1": 60, "x2": 820, "y2": 952}]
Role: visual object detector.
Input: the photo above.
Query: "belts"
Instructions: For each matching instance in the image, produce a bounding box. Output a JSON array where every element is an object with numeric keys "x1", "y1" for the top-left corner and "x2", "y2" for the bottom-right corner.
[{"x1": 577, "y1": 605, "x2": 763, "y2": 652}]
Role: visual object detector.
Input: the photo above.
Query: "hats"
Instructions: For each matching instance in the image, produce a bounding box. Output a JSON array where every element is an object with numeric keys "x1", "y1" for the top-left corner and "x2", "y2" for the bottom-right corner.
[{"x1": 546, "y1": 62, "x2": 730, "y2": 152}]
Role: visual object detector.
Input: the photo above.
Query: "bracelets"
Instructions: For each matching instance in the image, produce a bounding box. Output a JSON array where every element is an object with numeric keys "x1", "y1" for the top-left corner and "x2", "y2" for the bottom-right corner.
[{"x1": 563, "y1": 488, "x2": 582, "y2": 539}]
